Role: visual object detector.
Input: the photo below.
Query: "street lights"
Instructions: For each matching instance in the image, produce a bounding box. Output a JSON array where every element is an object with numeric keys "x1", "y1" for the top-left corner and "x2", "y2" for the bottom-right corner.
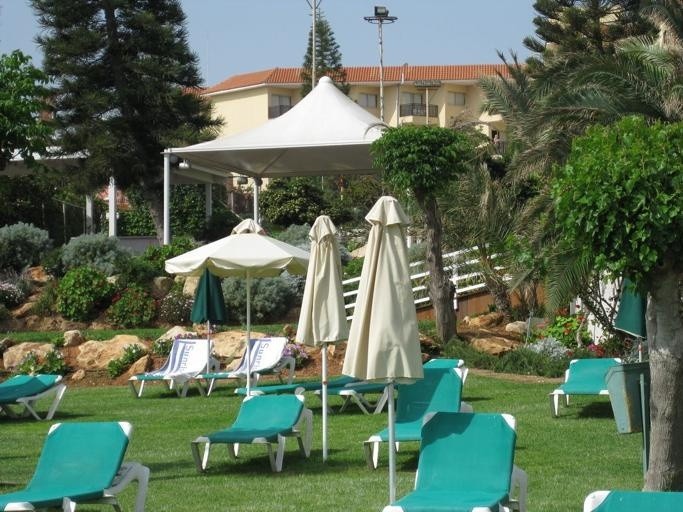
[{"x1": 364, "y1": 6, "x2": 398, "y2": 121}]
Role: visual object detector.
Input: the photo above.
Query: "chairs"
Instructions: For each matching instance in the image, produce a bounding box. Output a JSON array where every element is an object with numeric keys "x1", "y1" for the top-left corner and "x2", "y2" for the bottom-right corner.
[
  {"x1": 1, "y1": 422, "x2": 151, "y2": 512},
  {"x1": 364, "y1": 368, "x2": 464, "y2": 472},
  {"x1": 386, "y1": 411, "x2": 526, "y2": 512},
  {"x1": 191, "y1": 395, "x2": 313, "y2": 473},
  {"x1": 1, "y1": 374, "x2": 66, "y2": 421},
  {"x1": 197, "y1": 338, "x2": 297, "y2": 395},
  {"x1": 584, "y1": 490, "x2": 682, "y2": 512},
  {"x1": 130, "y1": 339, "x2": 220, "y2": 397}
]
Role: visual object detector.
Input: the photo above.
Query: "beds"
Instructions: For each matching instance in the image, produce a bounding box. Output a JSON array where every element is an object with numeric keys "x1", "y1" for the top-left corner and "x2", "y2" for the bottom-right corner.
[
  {"x1": 316, "y1": 358, "x2": 466, "y2": 416},
  {"x1": 550, "y1": 357, "x2": 623, "y2": 418},
  {"x1": 233, "y1": 374, "x2": 362, "y2": 408}
]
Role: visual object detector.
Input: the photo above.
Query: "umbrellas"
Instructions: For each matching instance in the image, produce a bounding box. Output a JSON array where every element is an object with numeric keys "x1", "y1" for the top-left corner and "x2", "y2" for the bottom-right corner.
[
  {"x1": 189, "y1": 269, "x2": 226, "y2": 374},
  {"x1": 165, "y1": 218, "x2": 310, "y2": 394},
  {"x1": 342, "y1": 196, "x2": 425, "y2": 507},
  {"x1": 294, "y1": 215, "x2": 348, "y2": 462}
]
